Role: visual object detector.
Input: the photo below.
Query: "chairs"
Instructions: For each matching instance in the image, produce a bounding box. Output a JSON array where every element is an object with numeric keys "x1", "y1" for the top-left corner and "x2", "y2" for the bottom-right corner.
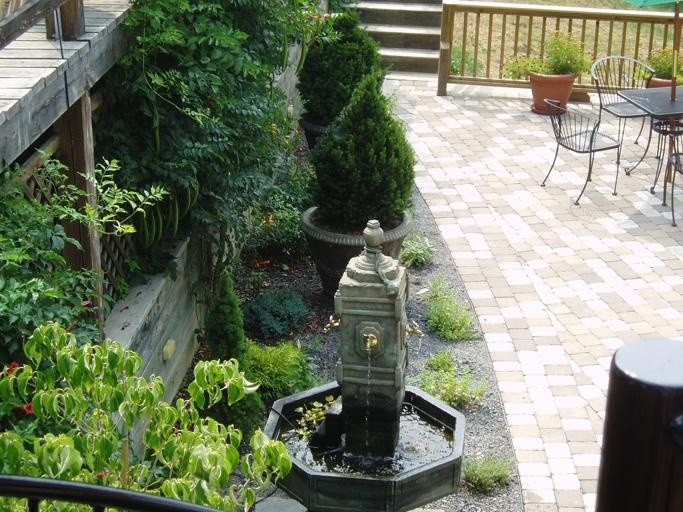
[{"x1": 541, "y1": 55, "x2": 683, "y2": 228}]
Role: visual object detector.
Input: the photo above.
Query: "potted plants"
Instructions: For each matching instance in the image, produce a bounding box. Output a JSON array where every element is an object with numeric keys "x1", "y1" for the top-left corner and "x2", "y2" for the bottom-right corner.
[
  {"x1": 639, "y1": 48, "x2": 680, "y2": 89},
  {"x1": 298, "y1": 10, "x2": 416, "y2": 298},
  {"x1": 502, "y1": 28, "x2": 592, "y2": 115}
]
